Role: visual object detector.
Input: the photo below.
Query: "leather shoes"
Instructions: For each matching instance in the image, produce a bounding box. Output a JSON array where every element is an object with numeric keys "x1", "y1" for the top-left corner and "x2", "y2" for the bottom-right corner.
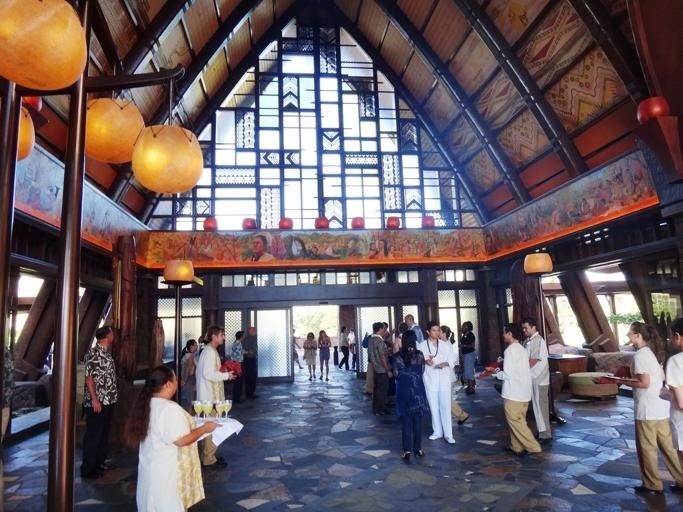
[
  {"x1": 313, "y1": 375, "x2": 316, "y2": 378},
  {"x1": 96, "y1": 462, "x2": 114, "y2": 470},
  {"x1": 214, "y1": 456, "x2": 224, "y2": 462},
  {"x1": 326, "y1": 378, "x2": 329, "y2": 381},
  {"x1": 309, "y1": 378, "x2": 312, "y2": 380},
  {"x1": 465, "y1": 385, "x2": 472, "y2": 391},
  {"x1": 319, "y1": 376, "x2": 322, "y2": 380},
  {"x1": 346, "y1": 368, "x2": 349, "y2": 370},
  {"x1": 669, "y1": 482, "x2": 682, "y2": 490},
  {"x1": 458, "y1": 413, "x2": 472, "y2": 425},
  {"x1": 505, "y1": 445, "x2": 526, "y2": 455},
  {"x1": 80, "y1": 471, "x2": 103, "y2": 479},
  {"x1": 468, "y1": 388, "x2": 475, "y2": 394},
  {"x1": 634, "y1": 486, "x2": 662, "y2": 494},
  {"x1": 338, "y1": 365, "x2": 342, "y2": 369},
  {"x1": 247, "y1": 395, "x2": 258, "y2": 400},
  {"x1": 535, "y1": 431, "x2": 553, "y2": 445},
  {"x1": 375, "y1": 411, "x2": 385, "y2": 416},
  {"x1": 232, "y1": 398, "x2": 244, "y2": 404},
  {"x1": 202, "y1": 462, "x2": 227, "y2": 469}
]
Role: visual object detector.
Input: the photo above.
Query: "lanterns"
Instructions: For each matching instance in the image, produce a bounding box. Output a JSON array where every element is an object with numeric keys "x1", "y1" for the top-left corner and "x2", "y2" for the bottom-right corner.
[
  {"x1": 84, "y1": 98, "x2": 144, "y2": 163},
  {"x1": 0, "y1": 0, "x2": 87, "y2": 90},
  {"x1": 132, "y1": 124, "x2": 203, "y2": 193},
  {"x1": 17, "y1": 107, "x2": 34, "y2": 160}
]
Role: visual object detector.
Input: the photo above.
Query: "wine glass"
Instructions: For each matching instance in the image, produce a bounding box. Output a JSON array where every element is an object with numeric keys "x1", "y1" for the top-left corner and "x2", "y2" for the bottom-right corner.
[
  {"x1": 215, "y1": 400, "x2": 224, "y2": 422},
  {"x1": 202, "y1": 400, "x2": 212, "y2": 423},
  {"x1": 192, "y1": 401, "x2": 202, "y2": 422},
  {"x1": 223, "y1": 399, "x2": 232, "y2": 423},
  {"x1": 617, "y1": 358, "x2": 631, "y2": 380}
]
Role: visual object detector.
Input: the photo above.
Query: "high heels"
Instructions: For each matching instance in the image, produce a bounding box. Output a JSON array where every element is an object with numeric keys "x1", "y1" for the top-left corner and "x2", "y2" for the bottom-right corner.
[
  {"x1": 414, "y1": 446, "x2": 424, "y2": 456},
  {"x1": 401, "y1": 450, "x2": 413, "y2": 460}
]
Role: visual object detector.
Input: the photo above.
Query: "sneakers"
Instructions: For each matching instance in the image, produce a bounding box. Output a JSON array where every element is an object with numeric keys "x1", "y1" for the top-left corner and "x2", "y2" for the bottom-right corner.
[
  {"x1": 428, "y1": 433, "x2": 444, "y2": 440},
  {"x1": 444, "y1": 436, "x2": 456, "y2": 444}
]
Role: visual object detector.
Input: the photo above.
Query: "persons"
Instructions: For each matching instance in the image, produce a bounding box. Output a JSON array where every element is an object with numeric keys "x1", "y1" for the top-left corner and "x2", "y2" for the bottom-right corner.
[
  {"x1": 125, "y1": 366, "x2": 224, "y2": 511},
  {"x1": 80, "y1": 325, "x2": 121, "y2": 478},
  {"x1": 241, "y1": 325, "x2": 261, "y2": 400},
  {"x1": 608, "y1": 322, "x2": 683, "y2": 494},
  {"x1": 350, "y1": 342, "x2": 358, "y2": 369},
  {"x1": 317, "y1": 330, "x2": 331, "y2": 381},
  {"x1": 338, "y1": 326, "x2": 351, "y2": 371},
  {"x1": 194, "y1": 324, "x2": 239, "y2": 472},
  {"x1": 491, "y1": 323, "x2": 543, "y2": 454},
  {"x1": 659, "y1": 317, "x2": 683, "y2": 469},
  {"x1": 476, "y1": 152, "x2": 658, "y2": 258},
  {"x1": 229, "y1": 331, "x2": 252, "y2": 405},
  {"x1": 360, "y1": 313, "x2": 477, "y2": 460},
  {"x1": 302, "y1": 331, "x2": 317, "y2": 382},
  {"x1": 520, "y1": 318, "x2": 554, "y2": 445},
  {"x1": 347, "y1": 328, "x2": 355, "y2": 354},
  {"x1": 293, "y1": 328, "x2": 304, "y2": 369},
  {"x1": 179, "y1": 338, "x2": 198, "y2": 416}
]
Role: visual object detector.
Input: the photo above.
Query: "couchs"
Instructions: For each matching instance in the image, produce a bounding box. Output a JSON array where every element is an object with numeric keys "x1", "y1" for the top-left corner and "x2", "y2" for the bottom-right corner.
[{"x1": 590, "y1": 350, "x2": 639, "y2": 398}]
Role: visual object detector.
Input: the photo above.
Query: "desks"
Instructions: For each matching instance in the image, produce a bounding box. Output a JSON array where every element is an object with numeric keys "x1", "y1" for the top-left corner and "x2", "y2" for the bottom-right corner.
[{"x1": 547, "y1": 353, "x2": 586, "y2": 378}]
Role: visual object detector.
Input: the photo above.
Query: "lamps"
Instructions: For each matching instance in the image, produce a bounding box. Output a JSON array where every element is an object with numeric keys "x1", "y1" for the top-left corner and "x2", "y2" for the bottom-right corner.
[
  {"x1": 203, "y1": 212, "x2": 436, "y2": 232},
  {"x1": 636, "y1": 95, "x2": 670, "y2": 124}
]
